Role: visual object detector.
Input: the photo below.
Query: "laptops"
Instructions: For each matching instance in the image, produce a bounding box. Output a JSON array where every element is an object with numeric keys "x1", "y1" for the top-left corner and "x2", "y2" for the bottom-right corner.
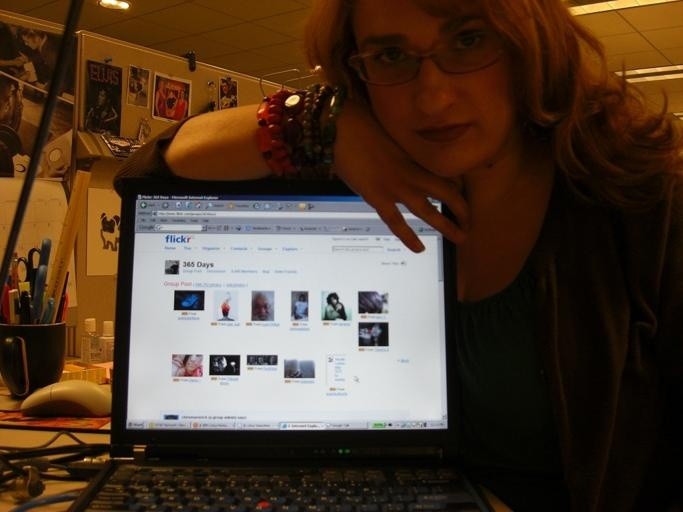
[{"x1": 69, "y1": 179, "x2": 496, "y2": 512}]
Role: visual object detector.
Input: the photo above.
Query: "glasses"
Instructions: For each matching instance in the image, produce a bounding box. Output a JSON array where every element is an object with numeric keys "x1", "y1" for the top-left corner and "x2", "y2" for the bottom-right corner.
[{"x1": 346, "y1": 30, "x2": 509, "y2": 87}]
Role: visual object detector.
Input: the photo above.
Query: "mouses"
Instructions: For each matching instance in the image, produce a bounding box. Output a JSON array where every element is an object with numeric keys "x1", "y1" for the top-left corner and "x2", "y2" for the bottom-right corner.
[{"x1": 21, "y1": 379, "x2": 110, "y2": 420}]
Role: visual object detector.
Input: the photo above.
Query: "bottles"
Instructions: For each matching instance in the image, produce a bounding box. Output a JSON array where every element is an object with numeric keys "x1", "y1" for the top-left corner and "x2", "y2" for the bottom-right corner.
[
  {"x1": 80, "y1": 317, "x2": 97, "y2": 363},
  {"x1": 97, "y1": 320, "x2": 115, "y2": 363}
]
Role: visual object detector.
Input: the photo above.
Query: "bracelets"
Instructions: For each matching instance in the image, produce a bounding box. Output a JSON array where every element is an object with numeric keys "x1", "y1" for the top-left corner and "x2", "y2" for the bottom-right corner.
[{"x1": 254, "y1": 85, "x2": 345, "y2": 181}]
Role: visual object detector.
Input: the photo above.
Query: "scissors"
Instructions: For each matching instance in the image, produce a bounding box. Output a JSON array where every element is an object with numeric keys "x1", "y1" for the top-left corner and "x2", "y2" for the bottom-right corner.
[
  {"x1": 33, "y1": 238, "x2": 51, "y2": 318},
  {"x1": 16, "y1": 248, "x2": 41, "y2": 299}
]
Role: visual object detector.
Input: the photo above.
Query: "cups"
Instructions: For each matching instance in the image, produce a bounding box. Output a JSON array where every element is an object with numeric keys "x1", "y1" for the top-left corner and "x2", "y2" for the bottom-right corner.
[{"x1": 0, "y1": 321, "x2": 66, "y2": 400}]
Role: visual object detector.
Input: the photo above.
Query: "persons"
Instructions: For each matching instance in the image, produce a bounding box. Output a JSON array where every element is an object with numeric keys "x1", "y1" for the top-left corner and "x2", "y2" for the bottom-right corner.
[
  {"x1": 323, "y1": 293, "x2": 347, "y2": 322},
  {"x1": 211, "y1": 356, "x2": 227, "y2": 375},
  {"x1": 170, "y1": 89, "x2": 188, "y2": 120},
  {"x1": 360, "y1": 323, "x2": 387, "y2": 346},
  {"x1": 1, "y1": 25, "x2": 74, "y2": 96},
  {"x1": 154, "y1": 79, "x2": 170, "y2": 120},
  {"x1": 172, "y1": 352, "x2": 203, "y2": 376},
  {"x1": 86, "y1": 88, "x2": 118, "y2": 124},
  {"x1": 219, "y1": 78, "x2": 235, "y2": 108},
  {"x1": 293, "y1": 295, "x2": 308, "y2": 320},
  {"x1": 112, "y1": 0, "x2": 683, "y2": 512},
  {"x1": 250, "y1": 293, "x2": 275, "y2": 321}
]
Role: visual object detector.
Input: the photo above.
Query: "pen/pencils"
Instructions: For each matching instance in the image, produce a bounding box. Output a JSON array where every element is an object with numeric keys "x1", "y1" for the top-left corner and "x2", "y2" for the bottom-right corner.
[{"x1": 3, "y1": 270, "x2": 71, "y2": 325}]
